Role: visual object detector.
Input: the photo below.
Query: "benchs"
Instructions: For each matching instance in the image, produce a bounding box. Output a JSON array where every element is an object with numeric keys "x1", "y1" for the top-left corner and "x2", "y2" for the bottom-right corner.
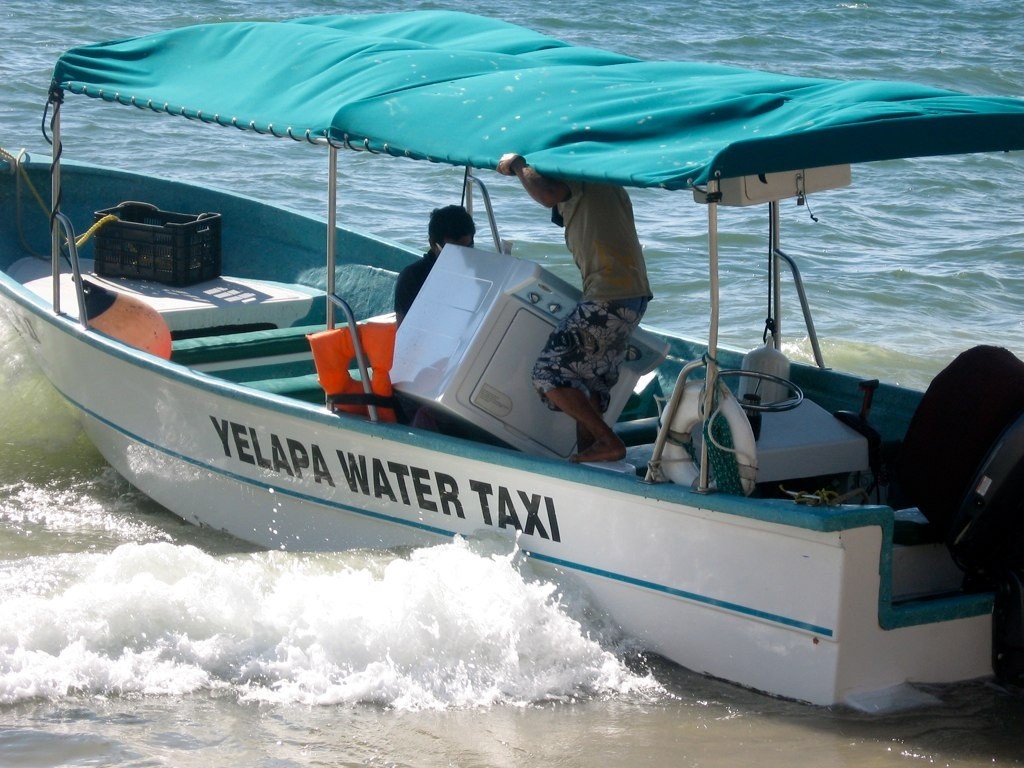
[
  {"x1": 170, "y1": 322, "x2": 378, "y2": 402},
  {"x1": 613, "y1": 413, "x2": 664, "y2": 477}
]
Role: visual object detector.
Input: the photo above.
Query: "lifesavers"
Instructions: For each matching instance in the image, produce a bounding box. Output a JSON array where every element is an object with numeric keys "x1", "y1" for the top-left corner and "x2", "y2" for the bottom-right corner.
[{"x1": 657, "y1": 379, "x2": 757, "y2": 495}]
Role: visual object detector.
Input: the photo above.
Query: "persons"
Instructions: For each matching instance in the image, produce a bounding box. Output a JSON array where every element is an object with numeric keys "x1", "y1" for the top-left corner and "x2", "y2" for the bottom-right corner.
[
  {"x1": 394, "y1": 206, "x2": 480, "y2": 434},
  {"x1": 495, "y1": 153, "x2": 653, "y2": 462}
]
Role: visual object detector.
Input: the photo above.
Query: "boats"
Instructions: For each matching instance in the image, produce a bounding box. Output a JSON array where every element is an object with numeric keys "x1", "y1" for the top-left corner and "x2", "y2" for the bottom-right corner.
[{"x1": 0, "y1": 8, "x2": 1024, "y2": 708}]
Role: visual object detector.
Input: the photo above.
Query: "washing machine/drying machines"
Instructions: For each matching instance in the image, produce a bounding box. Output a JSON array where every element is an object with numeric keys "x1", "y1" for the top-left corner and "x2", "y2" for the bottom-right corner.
[{"x1": 385, "y1": 235, "x2": 671, "y2": 468}]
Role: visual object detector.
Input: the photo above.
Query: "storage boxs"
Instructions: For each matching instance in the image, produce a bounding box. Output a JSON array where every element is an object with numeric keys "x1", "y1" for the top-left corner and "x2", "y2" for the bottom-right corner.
[{"x1": 92, "y1": 205, "x2": 222, "y2": 288}]
